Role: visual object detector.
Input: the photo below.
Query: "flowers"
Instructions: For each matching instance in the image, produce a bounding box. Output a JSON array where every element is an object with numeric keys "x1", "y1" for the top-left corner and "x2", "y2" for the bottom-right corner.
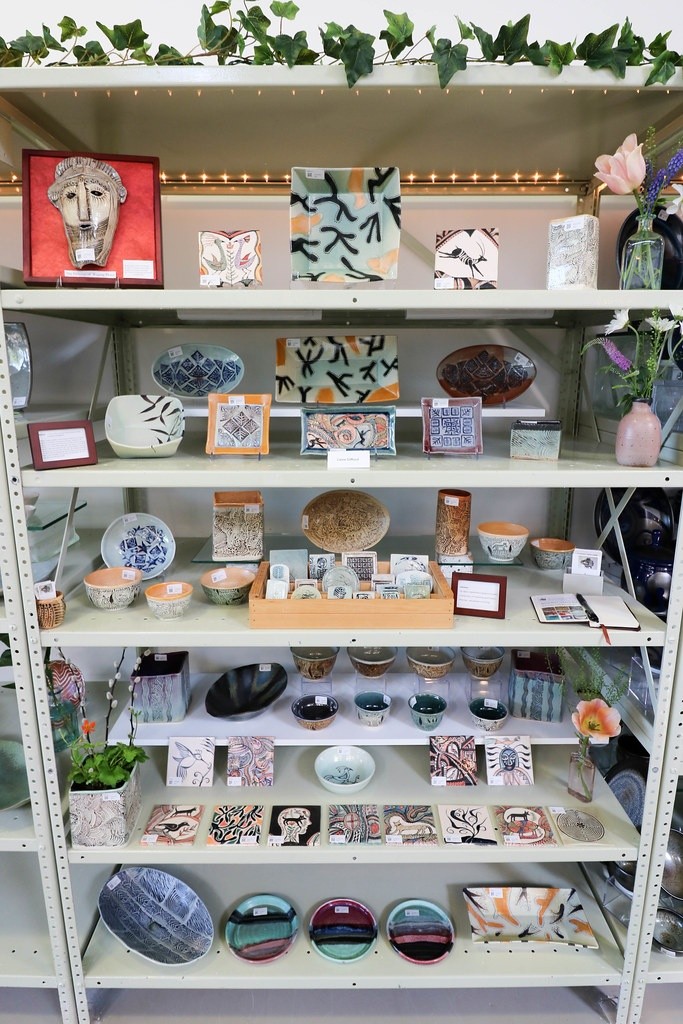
[
  {"x1": 580, "y1": 300, "x2": 682, "y2": 420},
  {"x1": 46, "y1": 646, "x2": 149, "y2": 789},
  {"x1": 593, "y1": 126, "x2": 683, "y2": 288},
  {"x1": 545, "y1": 647, "x2": 632, "y2": 797}
]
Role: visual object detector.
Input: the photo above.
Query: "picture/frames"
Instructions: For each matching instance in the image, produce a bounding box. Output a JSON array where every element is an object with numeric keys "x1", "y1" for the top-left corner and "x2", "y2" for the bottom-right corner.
[{"x1": 22, "y1": 148, "x2": 164, "y2": 289}]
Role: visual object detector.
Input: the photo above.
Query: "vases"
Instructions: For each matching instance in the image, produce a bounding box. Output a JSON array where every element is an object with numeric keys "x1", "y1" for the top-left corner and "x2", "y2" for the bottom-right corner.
[
  {"x1": 619, "y1": 214, "x2": 665, "y2": 290},
  {"x1": 67, "y1": 752, "x2": 143, "y2": 849},
  {"x1": 615, "y1": 398, "x2": 661, "y2": 467},
  {"x1": 568, "y1": 739, "x2": 596, "y2": 804}
]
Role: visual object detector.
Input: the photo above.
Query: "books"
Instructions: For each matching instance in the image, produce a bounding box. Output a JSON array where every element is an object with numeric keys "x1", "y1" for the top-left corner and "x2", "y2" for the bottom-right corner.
[{"x1": 529, "y1": 594, "x2": 641, "y2": 631}]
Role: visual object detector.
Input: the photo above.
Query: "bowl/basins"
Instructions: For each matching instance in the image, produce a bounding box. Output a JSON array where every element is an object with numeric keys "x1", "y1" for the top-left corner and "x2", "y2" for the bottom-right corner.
[
  {"x1": 145, "y1": 581, "x2": 193, "y2": 621},
  {"x1": 105, "y1": 394, "x2": 186, "y2": 457},
  {"x1": 84, "y1": 567, "x2": 142, "y2": 611},
  {"x1": 292, "y1": 648, "x2": 509, "y2": 730},
  {"x1": 96, "y1": 866, "x2": 214, "y2": 968},
  {"x1": 529, "y1": 538, "x2": 576, "y2": 569},
  {"x1": 201, "y1": 568, "x2": 255, "y2": 606},
  {"x1": 478, "y1": 522, "x2": 529, "y2": 562},
  {"x1": 204, "y1": 663, "x2": 288, "y2": 720},
  {"x1": 313, "y1": 745, "x2": 377, "y2": 794}
]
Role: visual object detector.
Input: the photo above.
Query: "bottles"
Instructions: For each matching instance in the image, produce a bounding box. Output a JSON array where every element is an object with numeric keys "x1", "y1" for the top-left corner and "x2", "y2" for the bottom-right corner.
[{"x1": 37, "y1": 591, "x2": 66, "y2": 629}]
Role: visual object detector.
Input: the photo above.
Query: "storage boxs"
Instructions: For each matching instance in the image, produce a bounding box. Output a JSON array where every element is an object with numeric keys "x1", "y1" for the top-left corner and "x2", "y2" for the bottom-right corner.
[{"x1": 249, "y1": 561, "x2": 454, "y2": 630}]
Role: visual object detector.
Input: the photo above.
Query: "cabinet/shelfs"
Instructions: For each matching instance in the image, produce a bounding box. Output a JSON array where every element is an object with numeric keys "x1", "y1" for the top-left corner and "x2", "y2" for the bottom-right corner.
[{"x1": 0, "y1": 66, "x2": 683, "y2": 1024}]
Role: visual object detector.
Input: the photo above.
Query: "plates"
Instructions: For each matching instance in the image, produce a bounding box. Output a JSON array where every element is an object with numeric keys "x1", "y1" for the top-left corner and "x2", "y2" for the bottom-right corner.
[
  {"x1": 420, "y1": 396, "x2": 483, "y2": 455},
  {"x1": 462, "y1": 886, "x2": 598, "y2": 949},
  {"x1": 290, "y1": 165, "x2": 401, "y2": 284},
  {"x1": 301, "y1": 490, "x2": 390, "y2": 553},
  {"x1": 5, "y1": 323, "x2": 32, "y2": 411},
  {"x1": 224, "y1": 895, "x2": 454, "y2": 964},
  {"x1": 151, "y1": 343, "x2": 244, "y2": 400},
  {"x1": 300, "y1": 407, "x2": 396, "y2": 456},
  {"x1": 101, "y1": 513, "x2": 176, "y2": 581},
  {"x1": 204, "y1": 394, "x2": 274, "y2": 454},
  {"x1": 0, "y1": 740, "x2": 30, "y2": 811},
  {"x1": 435, "y1": 344, "x2": 536, "y2": 405},
  {"x1": 274, "y1": 334, "x2": 401, "y2": 405}
]
